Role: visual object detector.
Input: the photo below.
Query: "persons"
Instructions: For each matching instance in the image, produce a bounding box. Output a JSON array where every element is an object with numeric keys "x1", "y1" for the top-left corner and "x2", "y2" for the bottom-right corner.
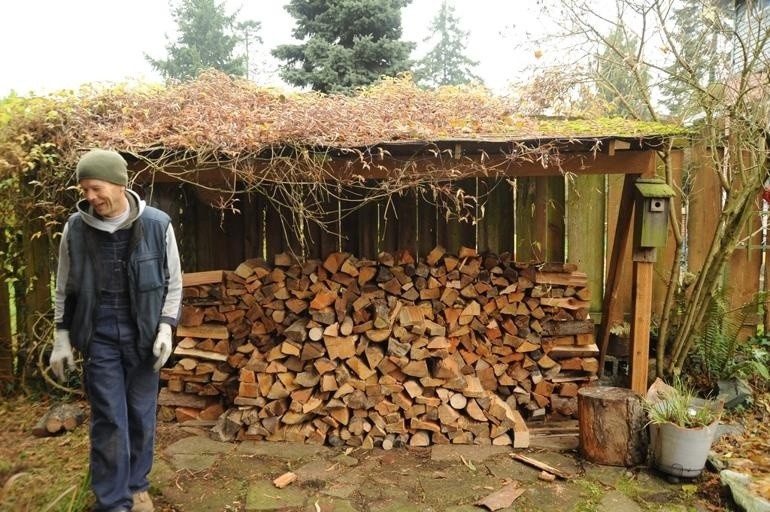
[{"x1": 50, "y1": 150, "x2": 183, "y2": 512}]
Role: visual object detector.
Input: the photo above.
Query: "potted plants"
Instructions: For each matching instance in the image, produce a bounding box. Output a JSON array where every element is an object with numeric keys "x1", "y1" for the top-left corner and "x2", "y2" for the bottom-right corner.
[{"x1": 637, "y1": 368, "x2": 723, "y2": 480}]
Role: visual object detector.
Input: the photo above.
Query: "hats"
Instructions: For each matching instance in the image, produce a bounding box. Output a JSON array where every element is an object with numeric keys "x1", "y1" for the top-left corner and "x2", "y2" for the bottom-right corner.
[{"x1": 76, "y1": 150, "x2": 128, "y2": 185}]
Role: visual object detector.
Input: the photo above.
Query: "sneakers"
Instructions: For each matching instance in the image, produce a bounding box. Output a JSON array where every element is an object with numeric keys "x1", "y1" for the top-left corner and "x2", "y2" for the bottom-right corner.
[{"x1": 132, "y1": 491, "x2": 155, "y2": 512}]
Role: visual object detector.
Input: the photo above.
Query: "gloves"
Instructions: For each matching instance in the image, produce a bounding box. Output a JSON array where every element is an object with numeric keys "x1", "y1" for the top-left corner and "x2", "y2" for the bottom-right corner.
[
  {"x1": 153, "y1": 323, "x2": 172, "y2": 372},
  {"x1": 50, "y1": 331, "x2": 77, "y2": 382}
]
